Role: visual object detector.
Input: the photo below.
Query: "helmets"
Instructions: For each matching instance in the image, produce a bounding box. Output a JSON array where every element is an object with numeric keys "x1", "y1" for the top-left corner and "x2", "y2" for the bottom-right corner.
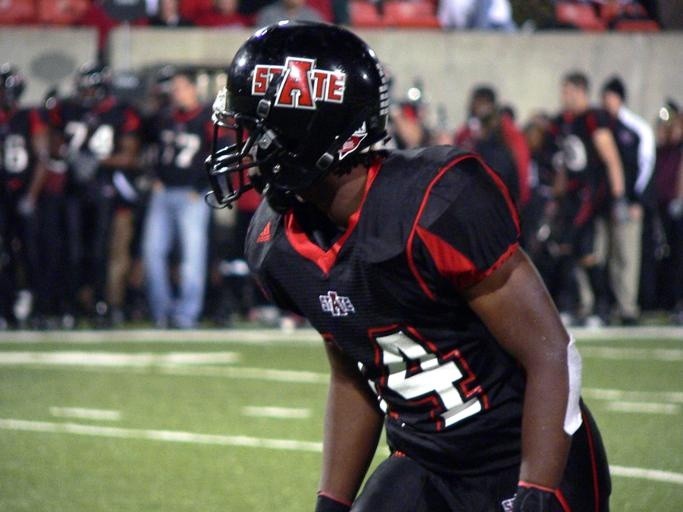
[{"x1": 225, "y1": 18, "x2": 390, "y2": 216}]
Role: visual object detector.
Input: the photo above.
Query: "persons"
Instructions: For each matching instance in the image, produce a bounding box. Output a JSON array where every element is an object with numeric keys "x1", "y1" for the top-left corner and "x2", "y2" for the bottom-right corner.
[{"x1": 204, "y1": 17, "x2": 615, "y2": 510}]
